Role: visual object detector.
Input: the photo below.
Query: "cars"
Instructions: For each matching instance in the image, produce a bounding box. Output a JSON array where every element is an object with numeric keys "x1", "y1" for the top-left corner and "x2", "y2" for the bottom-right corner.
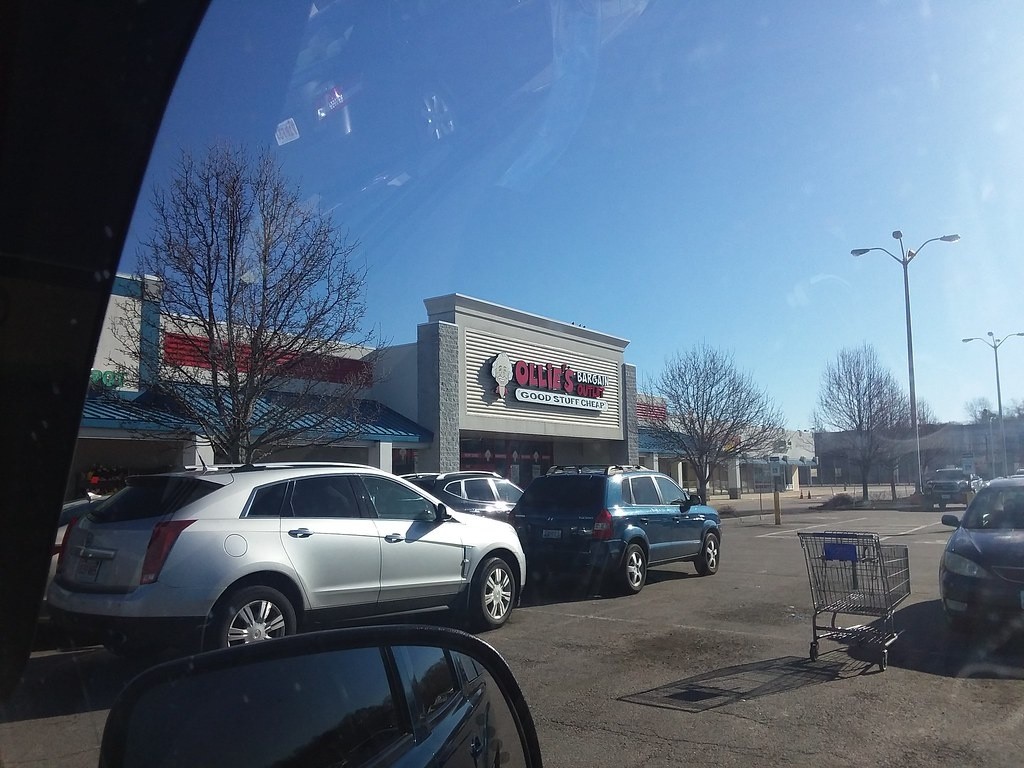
[
  {"x1": 940, "y1": 474, "x2": 1024, "y2": 635},
  {"x1": 45, "y1": 488, "x2": 114, "y2": 603}
]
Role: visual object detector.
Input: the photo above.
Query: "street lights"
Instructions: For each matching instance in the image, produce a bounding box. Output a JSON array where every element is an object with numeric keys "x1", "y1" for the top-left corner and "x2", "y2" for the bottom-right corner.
[
  {"x1": 851, "y1": 230, "x2": 961, "y2": 494},
  {"x1": 962, "y1": 332, "x2": 1024, "y2": 475}
]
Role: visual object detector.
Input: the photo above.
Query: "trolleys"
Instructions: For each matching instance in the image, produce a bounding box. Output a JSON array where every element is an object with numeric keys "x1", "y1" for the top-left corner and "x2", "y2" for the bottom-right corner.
[{"x1": 797, "y1": 528, "x2": 911, "y2": 671}]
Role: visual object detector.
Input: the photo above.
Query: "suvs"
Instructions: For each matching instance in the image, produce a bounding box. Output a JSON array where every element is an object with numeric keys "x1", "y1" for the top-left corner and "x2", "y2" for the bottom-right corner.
[
  {"x1": 509, "y1": 462, "x2": 721, "y2": 594},
  {"x1": 924, "y1": 467, "x2": 981, "y2": 505},
  {"x1": 394, "y1": 471, "x2": 525, "y2": 522},
  {"x1": 44, "y1": 460, "x2": 526, "y2": 661}
]
